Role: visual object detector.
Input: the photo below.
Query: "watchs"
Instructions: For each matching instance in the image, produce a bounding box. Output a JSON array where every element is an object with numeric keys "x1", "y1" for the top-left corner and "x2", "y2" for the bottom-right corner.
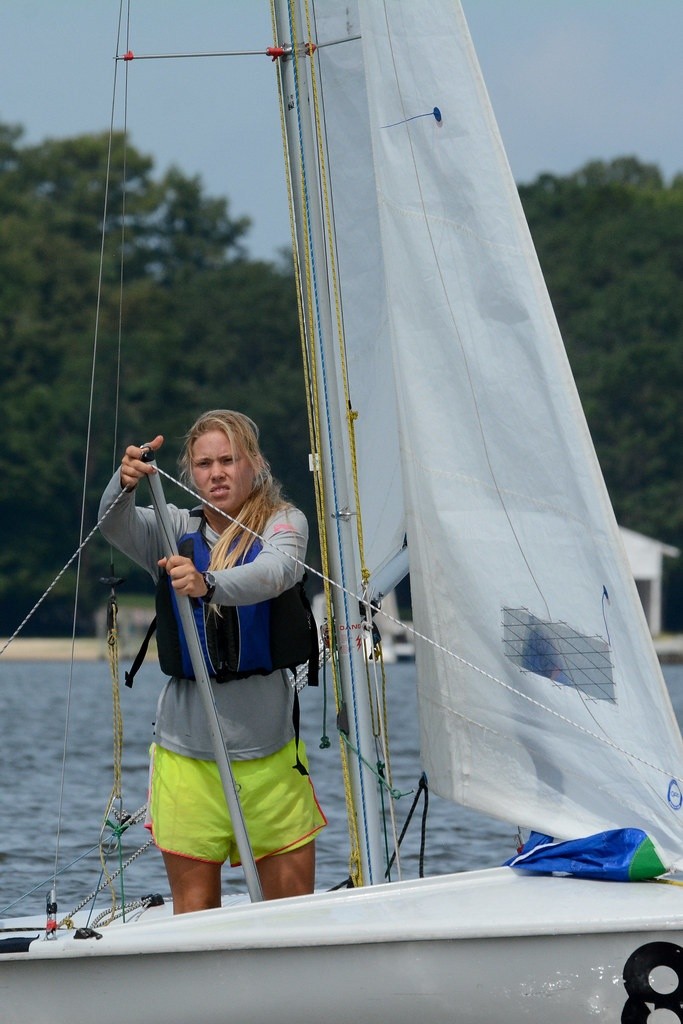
[{"x1": 199, "y1": 570, "x2": 216, "y2": 600}]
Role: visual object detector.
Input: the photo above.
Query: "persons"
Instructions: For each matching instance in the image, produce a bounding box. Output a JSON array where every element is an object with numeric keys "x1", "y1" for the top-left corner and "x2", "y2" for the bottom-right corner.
[{"x1": 97, "y1": 410, "x2": 327, "y2": 915}]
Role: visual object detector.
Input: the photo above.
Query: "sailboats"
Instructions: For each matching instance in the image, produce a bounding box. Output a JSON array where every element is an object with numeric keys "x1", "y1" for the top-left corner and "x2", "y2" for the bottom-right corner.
[{"x1": 1, "y1": 0, "x2": 683, "y2": 1023}]
